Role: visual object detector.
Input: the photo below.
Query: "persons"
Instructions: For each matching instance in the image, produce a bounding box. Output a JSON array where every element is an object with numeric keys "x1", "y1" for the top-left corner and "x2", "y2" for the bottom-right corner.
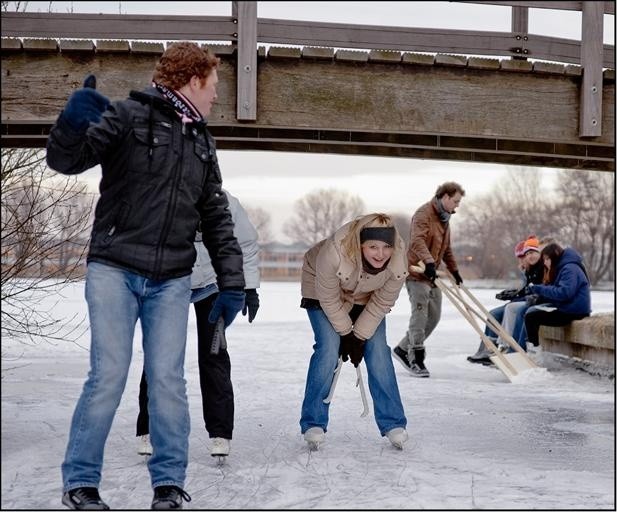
[
  {"x1": 466, "y1": 240, "x2": 529, "y2": 366},
  {"x1": 388, "y1": 181, "x2": 465, "y2": 379},
  {"x1": 44, "y1": 37, "x2": 247, "y2": 512},
  {"x1": 134, "y1": 184, "x2": 261, "y2": 458},
  {"x1": 298, "y1": 210, "x2": 411, "y2": 446},
  {"x1": 522, "y1": 241, "x2": 593, "y2": 354},
  {"x1": 495, "y1": 232, "x2": 543, "y2": 355}
]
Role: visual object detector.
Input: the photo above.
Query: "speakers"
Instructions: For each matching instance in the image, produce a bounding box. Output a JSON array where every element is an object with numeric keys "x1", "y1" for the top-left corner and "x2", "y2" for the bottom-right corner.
[{"x1": 525, "y1": 342, "x2": 544, "y2": 367}]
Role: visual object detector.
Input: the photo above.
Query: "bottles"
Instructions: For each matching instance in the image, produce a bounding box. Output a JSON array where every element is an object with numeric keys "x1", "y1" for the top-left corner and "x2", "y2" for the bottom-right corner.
[
  {"x1": 523, "y1": 238, "x2": 542, "y2": 254},
  {"x1": 514, "y1": 241, "x2": 525, "y2": 258}
]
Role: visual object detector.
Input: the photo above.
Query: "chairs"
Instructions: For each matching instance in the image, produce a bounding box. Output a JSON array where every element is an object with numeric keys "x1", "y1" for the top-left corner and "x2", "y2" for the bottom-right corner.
[
  {"x1": 61, "y1": 487, "x2": 111, "y2": 510},
  {"x1": 136, "y1": 433, "x2": 153, "y2": 456},
  {"x1": 391, "y1": 345, "x2": 410, "y2": 371},
  {"x1": 408, "y1": 359, "x2": 430, "y2": 378},
  {"x1": 303, "y1": 426, "x2": 325, "y2": 443},
  {"x1": 151, "y1": 486, "x2": 184, "y2": 510},
  {"x1": 209, "y1": 436, "x2": 231, "y2": 457},
  {"x1": 385, "y1": 427, "x2": 409, "y2": 445},
  {"x1": 467, "y1": 346, "x2": 513, "y2": 366}
]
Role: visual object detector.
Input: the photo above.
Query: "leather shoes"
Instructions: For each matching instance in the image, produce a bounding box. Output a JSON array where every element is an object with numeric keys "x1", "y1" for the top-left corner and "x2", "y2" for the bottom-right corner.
[
  {"x1": 495, "y1": 284, "x2": 543, "y2": 306},
  {"x1": 338, "y1": 329, "x2": 353, "y2": 363},
  {"x1": 62, "y1": 73, "x2": 111, "y2": 129},
  {"x1": 208, "y1": 288, "x2": 248, "y2": 333},
  {"x1": 348, "y1": 333, "x2": 366, "y2": 368},
  {"x1": 423, "y1": 263, "x2": 439, "y2": 284},
  {"x1": 242, "y1": 290, "x2": 260, "y2": 323},
  {"x1": 451, "y1": 270, "x2": 463, "y2": 286}
]
